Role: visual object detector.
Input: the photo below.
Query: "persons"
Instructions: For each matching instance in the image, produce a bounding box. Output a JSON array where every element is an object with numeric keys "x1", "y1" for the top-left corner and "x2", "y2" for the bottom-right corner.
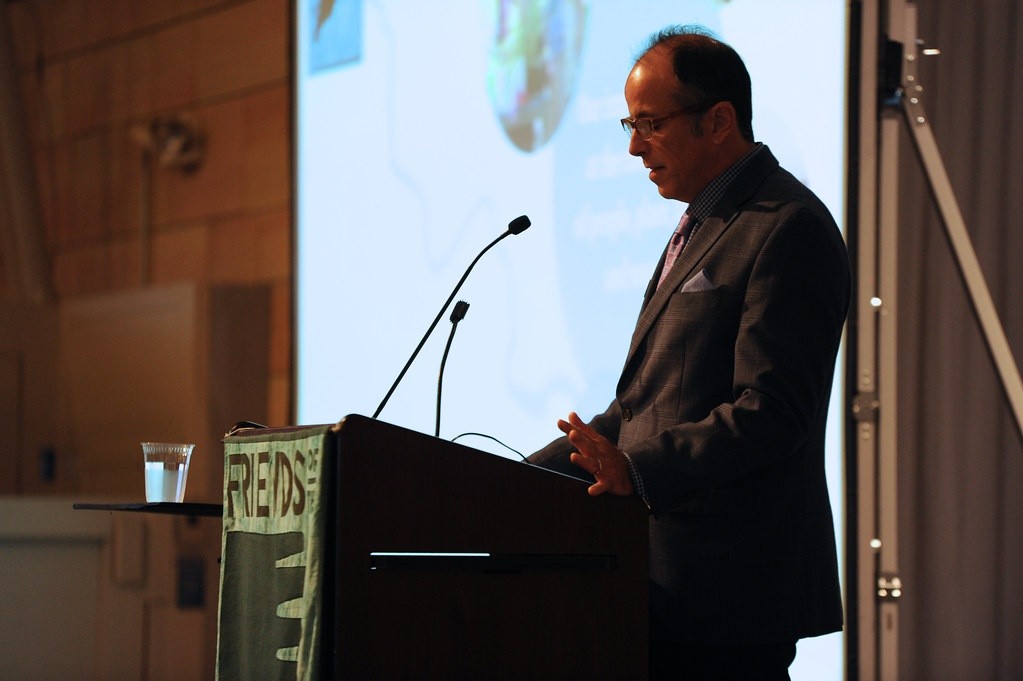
[{"x1": 519, "y1": 24, "x2": 853, "y2": 680}]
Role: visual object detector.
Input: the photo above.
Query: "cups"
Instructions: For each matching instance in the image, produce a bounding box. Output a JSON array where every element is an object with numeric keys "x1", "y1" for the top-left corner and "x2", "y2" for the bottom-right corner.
[{"x1": 140, "y1": 443, "x2": 195, "y2": 503}]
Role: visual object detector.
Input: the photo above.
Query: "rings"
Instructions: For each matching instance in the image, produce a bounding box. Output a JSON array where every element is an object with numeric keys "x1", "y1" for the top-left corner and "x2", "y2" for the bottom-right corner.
[{"x1": 598, "y1": 459, "x2": 602, "y2": 472}]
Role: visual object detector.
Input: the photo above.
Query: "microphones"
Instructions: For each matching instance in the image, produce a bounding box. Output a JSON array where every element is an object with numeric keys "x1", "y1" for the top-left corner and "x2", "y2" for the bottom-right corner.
[
  {"x1": 372, "y1": 215, "x2": 531, "y2": 420},
  {"x1": 443, "y1": 301, "x2": 470, "y2": 441}
]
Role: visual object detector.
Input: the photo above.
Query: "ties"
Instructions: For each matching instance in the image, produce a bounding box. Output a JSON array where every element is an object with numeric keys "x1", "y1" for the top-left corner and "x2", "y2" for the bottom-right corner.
[{"x1": 657, "y1": 210, "x2": 698, "y2": 291}]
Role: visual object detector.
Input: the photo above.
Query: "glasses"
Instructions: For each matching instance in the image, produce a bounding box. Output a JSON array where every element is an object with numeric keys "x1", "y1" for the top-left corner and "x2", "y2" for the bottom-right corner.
[{"x1": 621, "y1": 100, "x2": 715, "y2": 139}]
